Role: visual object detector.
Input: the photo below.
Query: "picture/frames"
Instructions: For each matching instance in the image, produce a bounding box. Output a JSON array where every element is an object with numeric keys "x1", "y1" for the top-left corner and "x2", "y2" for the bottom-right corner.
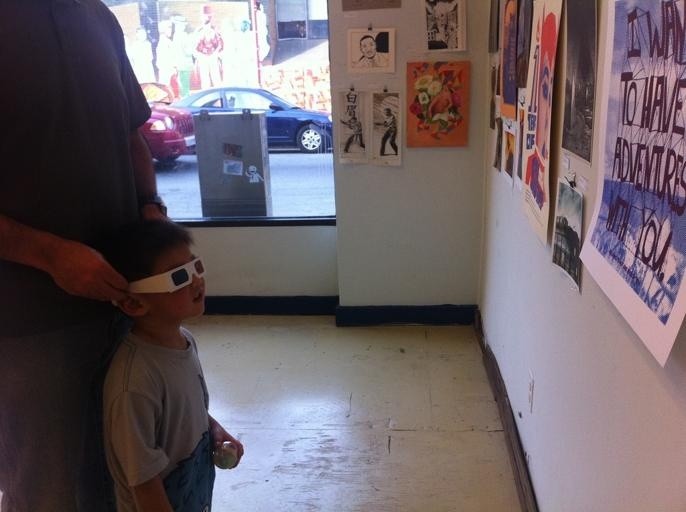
[
  {"x1": 347, "y1": 27, "x2": 396, "y2": 74},
  {"x1": 420, "y1": 0, "x2": 467, "y2": 52}
]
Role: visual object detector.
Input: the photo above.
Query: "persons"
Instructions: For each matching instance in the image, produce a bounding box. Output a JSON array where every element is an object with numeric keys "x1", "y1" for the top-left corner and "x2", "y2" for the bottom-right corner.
[
  {"x1": 340, "y1": 116, "x2": 366, "y2": 153},
  {"x1": 1, "y1": 1, "x2": 167, "y2": 512},
  {"x1": 354, "y1": 34, "x2": 388, "y2": 67},
  {"x1": 375, "y1": 107, "x2": 399, "y2": 156},
  {"x1": 101, "y1": 217, "x2": 245, "y2": 512}
]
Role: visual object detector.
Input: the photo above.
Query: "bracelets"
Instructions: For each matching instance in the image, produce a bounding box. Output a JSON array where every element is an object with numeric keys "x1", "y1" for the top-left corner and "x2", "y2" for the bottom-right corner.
[{"x1": 137, "y1": 193, "x2": 167, "y2": 215}]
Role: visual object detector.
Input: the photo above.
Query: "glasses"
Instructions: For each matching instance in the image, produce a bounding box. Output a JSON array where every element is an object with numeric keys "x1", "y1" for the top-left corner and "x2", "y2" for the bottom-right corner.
[{"x1": 126, "y1": 258, "x2": 206, "y2": 296}]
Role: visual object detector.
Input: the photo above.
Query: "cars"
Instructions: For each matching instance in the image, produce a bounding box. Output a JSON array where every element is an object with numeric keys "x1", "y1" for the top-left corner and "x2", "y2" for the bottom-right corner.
[
  {"x1": 142, "y1": 101, "x2": 195, "y2": 161},
  {"x1": 182, "y1": 89, "x2": 331, "y2": 150}
]
